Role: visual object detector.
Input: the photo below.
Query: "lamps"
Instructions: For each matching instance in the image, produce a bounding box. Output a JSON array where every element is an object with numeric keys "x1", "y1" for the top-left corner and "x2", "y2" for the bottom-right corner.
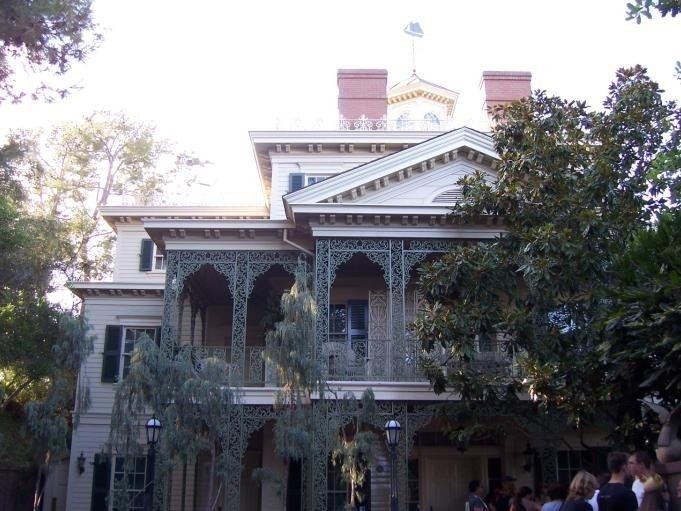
[{"x1": 77, "y1": 450, "x2": 87, "y2": 474}]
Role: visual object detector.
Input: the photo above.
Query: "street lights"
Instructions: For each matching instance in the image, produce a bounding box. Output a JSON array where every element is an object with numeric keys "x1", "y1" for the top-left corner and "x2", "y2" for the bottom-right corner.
[
  {"x1": 385, "y1": 419, "x2": 401, "y2": 511},
  {"x1": 145, "y1": 414, "x2": 162, "y2": 510}
]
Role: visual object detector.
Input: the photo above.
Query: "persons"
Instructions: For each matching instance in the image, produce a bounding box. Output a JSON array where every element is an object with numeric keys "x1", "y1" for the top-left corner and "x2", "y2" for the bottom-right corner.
[{"x1": 468, "y1": 450, "x2": 671, "y2": 511}]
